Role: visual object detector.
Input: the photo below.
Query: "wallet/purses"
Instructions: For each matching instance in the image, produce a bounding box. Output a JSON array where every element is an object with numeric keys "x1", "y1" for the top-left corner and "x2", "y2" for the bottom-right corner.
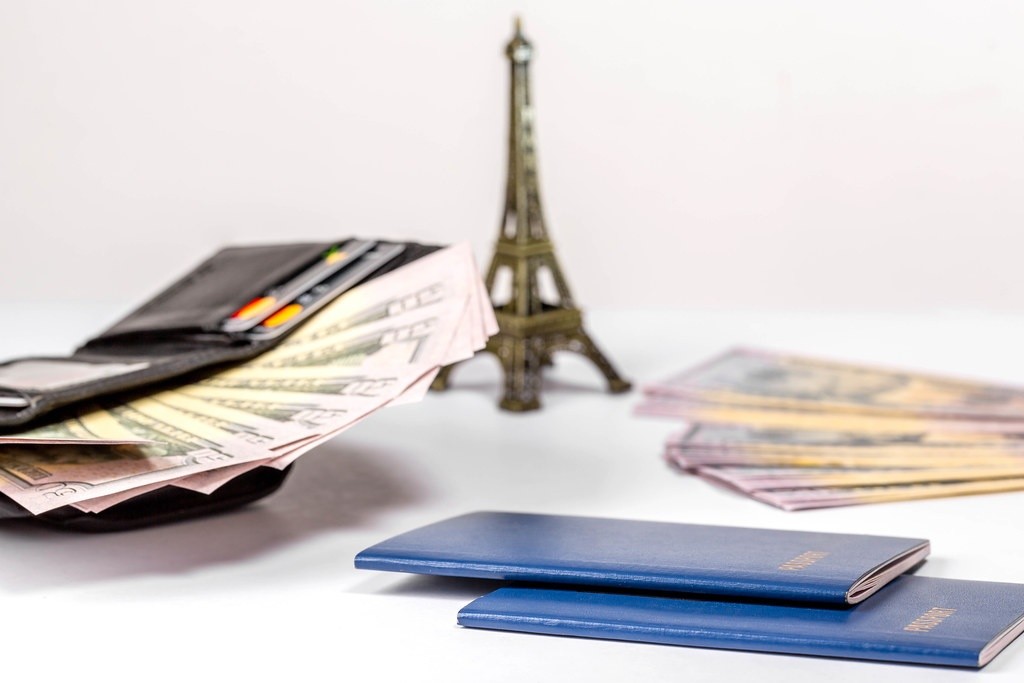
[{"x1": 0, "y1": 238, "x2": 445, "y2": 531}]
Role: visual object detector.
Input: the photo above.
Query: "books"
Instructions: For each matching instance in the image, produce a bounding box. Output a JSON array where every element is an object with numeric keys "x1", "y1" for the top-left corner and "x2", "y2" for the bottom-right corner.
[
  {"x1": 354, "y1": 511, "x2": 931, "y2": 605},
  {"x1": 457, "y1": 575, "x2": 1024, "y2": 667}
]
